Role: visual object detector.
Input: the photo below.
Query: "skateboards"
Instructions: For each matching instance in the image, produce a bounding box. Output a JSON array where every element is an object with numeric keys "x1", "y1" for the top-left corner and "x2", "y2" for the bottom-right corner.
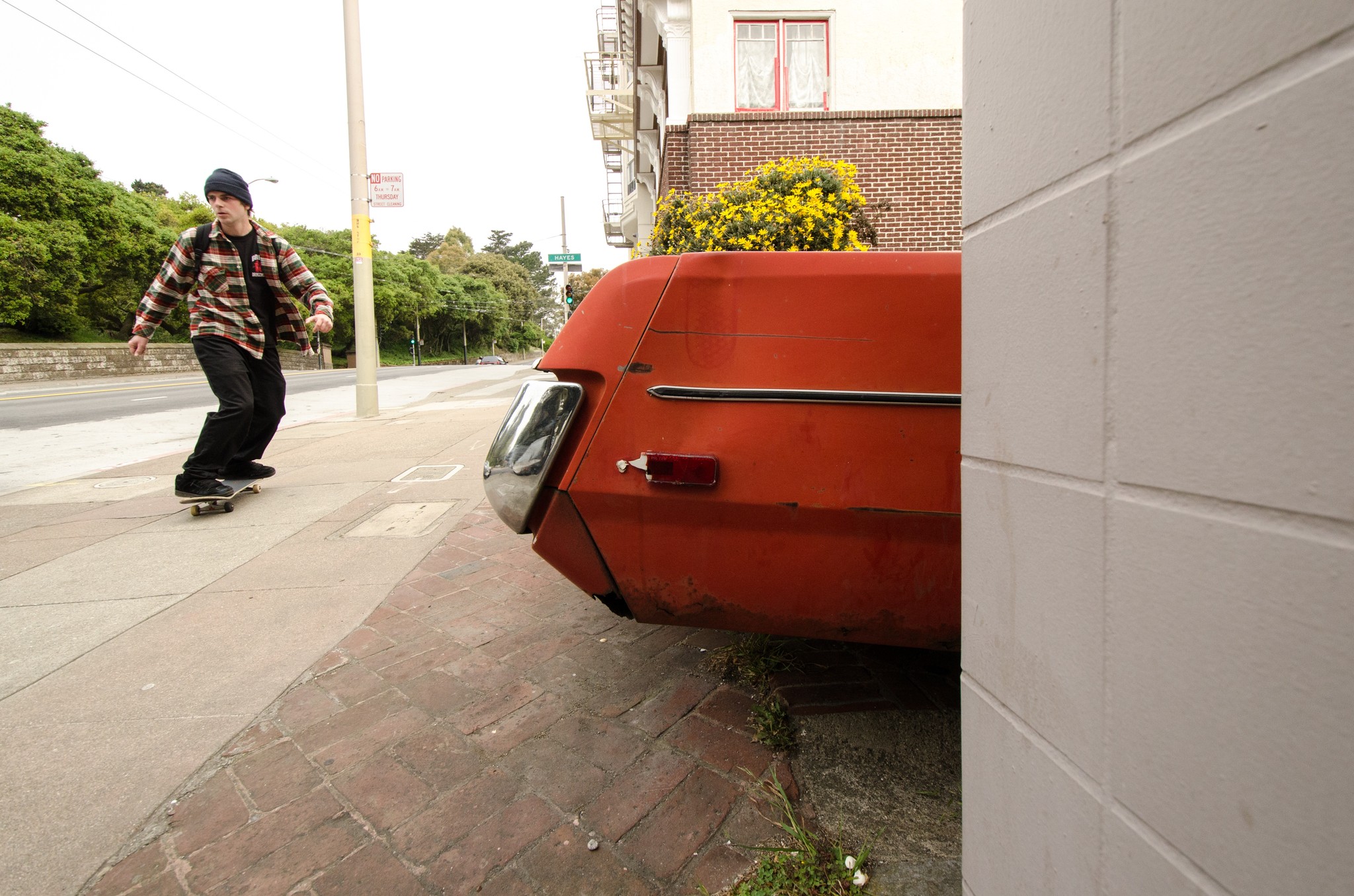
[{"x1": 176, "y1": 463, "x2": 270, "y2": 518}]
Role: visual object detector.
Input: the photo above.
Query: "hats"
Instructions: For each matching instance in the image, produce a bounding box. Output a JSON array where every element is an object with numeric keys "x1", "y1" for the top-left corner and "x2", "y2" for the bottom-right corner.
[{"x1": 204, "y1": 169, "x2": 254, "y2": 205}]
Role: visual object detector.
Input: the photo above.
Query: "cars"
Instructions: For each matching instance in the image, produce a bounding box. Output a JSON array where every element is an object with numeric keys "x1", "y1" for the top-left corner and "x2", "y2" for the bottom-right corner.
[
  {"x1": 480, "y1": 246, "x2": 962, "y2": 656},
  {"x1": 479, "y1": 355, "x2": 508, "y2": 365}
]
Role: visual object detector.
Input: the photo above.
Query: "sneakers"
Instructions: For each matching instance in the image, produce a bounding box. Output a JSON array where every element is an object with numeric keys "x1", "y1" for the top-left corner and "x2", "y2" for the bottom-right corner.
[
  {"x1": 174, "y1": 474, "x2": 234, "y2": 498},
  {"x1": 216, "y1": 461, "x2": 275, "y2": 481}
]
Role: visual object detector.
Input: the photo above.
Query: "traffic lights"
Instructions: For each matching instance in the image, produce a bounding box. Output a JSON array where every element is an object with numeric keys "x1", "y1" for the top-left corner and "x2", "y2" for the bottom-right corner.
[{"x1": 565, "y1": 284, "x2": 573, "y2": 304}]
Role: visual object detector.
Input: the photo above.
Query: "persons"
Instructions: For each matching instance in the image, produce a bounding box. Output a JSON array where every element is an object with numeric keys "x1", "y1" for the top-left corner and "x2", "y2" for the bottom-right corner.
[{"x1": 129, "y1": 168, "x2": 335, "y2": 499}]
